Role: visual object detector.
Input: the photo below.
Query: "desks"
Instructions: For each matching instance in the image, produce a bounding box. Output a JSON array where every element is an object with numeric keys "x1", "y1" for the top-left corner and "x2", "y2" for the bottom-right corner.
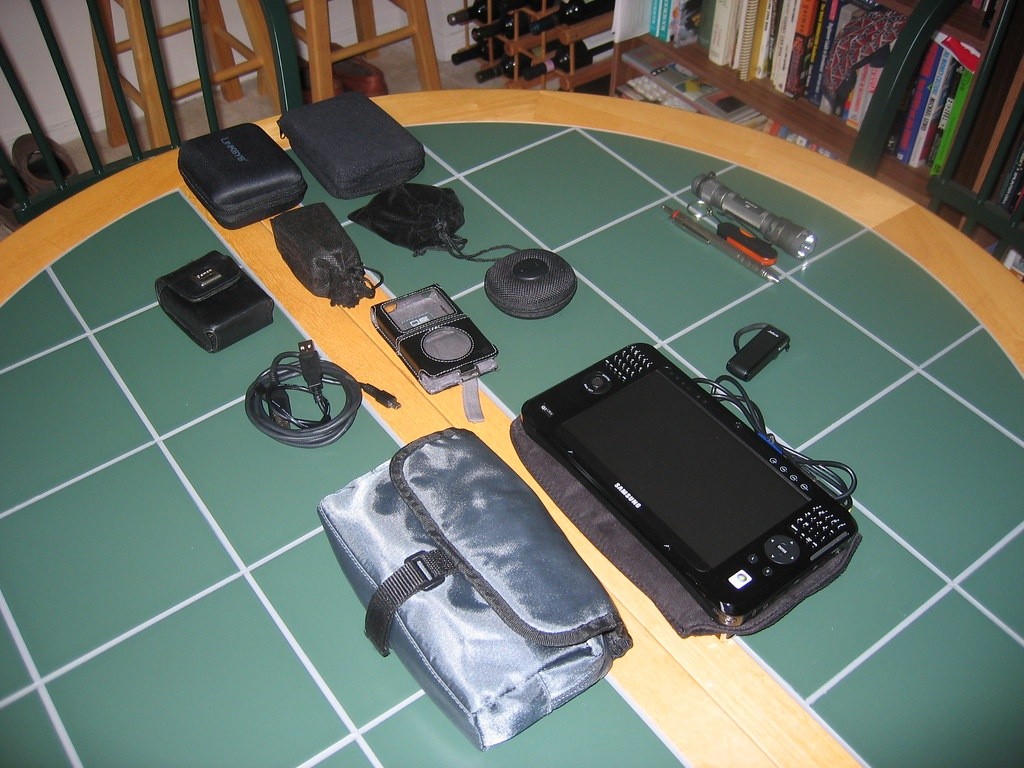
[{"x1": 1, "y1": 90, "x2": 1022, "y2": 767}]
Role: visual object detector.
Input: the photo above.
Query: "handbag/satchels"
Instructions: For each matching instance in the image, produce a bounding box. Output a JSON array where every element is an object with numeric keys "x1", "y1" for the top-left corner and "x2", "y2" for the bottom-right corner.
[{"x1": 316, "y1": 426, "x2": 633, "y2": 753}]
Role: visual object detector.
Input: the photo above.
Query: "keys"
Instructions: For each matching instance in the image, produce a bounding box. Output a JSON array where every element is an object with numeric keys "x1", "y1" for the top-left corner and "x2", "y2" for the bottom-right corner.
[{"x1": 725, "y1": 321, "x2": 792, "y2": 382}]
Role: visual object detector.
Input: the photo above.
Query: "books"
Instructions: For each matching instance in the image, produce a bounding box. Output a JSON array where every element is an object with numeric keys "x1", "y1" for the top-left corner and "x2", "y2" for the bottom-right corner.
[
  {"x1": 616, "y1": 43, "x2": 847, "y2": 165},
  {"x1": 966, "y1": 0, "x2": 999, "y2": 29},
  {"x1": 611, "y1": 0, "x2": 909, "y2": 131},
  {"x1": 990, "y1": 118, "x2": 1024, "y2": 224},
  {"x1": 887, "y1": 30, "x2": 982, "y2": 174}
]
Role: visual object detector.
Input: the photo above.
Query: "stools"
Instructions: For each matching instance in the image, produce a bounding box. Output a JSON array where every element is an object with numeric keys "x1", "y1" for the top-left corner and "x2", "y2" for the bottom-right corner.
[{"x1": 87, "y1": 0, "x2": 442, "y2": 151}]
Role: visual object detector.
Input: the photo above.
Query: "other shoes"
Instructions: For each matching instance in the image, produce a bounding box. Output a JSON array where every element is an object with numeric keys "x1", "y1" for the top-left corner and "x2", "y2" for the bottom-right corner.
[
  {"x1": 12, "y1": 133, "x2": 78, "y2": 198},
  {"x1": 0, "y1": 169, "x2": 44, "y2": 234}
]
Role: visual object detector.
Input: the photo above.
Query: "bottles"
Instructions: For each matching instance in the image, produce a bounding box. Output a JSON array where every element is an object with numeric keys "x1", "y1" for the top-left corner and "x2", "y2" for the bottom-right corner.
[{"x1": 447, "y1": 0, "x2": 616, "y2": 96}]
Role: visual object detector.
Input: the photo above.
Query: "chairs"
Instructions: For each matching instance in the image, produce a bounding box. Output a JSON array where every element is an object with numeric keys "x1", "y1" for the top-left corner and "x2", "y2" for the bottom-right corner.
[{"x1": 845, "y1": 0, "x2": 1024, "y2": 277}]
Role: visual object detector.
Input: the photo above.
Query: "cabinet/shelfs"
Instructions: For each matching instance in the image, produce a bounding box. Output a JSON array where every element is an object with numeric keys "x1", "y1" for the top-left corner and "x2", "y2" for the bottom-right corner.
[{"x1": 609, "y1": 0, "x2": 1024, "y2": 280}]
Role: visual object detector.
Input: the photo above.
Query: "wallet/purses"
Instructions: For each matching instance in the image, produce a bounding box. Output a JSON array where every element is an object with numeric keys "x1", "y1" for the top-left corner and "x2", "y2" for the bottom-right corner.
[
  {"x1": 276, "y1": 92, "x2": 425, "y2": 201},
  {"x1": 178, "y1": 124, "x2": 308, "y2": 230}
]
